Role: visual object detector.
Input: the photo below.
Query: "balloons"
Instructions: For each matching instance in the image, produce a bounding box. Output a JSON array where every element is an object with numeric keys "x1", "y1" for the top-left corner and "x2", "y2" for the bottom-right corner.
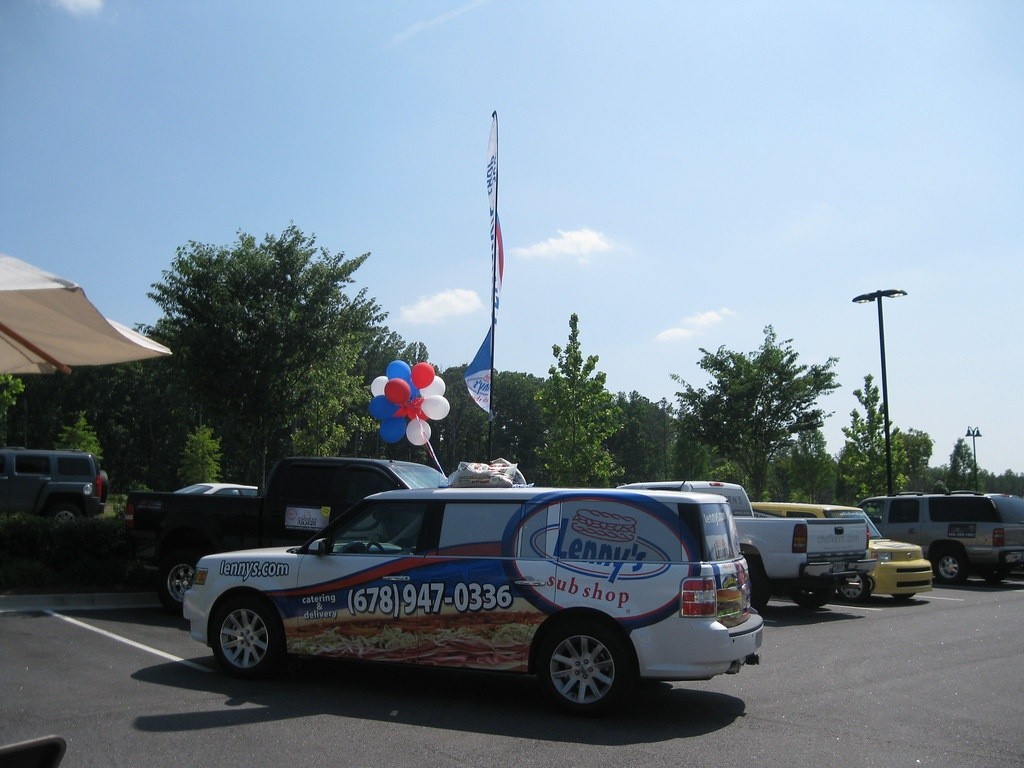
[
  {"x1": 420, "y1": 395, "x2": 450, "y2": 421},
  {"x1": 386, "y1": 360, "x2": 411, "y2": 384},
  {"x1": 418, "y1": 375, "x2": 446, "y2": 400},
  {"x1": 379, "y1": 416, "x2": 408, "y2": 444},
  {"x1": 405, "y1": 417, "x2": 431, "y2": 446},
  {"x1": 367, "y1": 395, "x2": 401, "y2": 420},
  {"x1": 407, "y1": 397, "x2": 430, "y2": 422},
  {"x1": 384, "y1": 378, "x2": 411, "y2": 405},
  {"x1": 390, "y1": 401, "x2": 410, "y2": 419},
  {"x1": 370, "y1": 375, "x2": 390, "y2": 398},
  {"x1": 401, "y1": 375, "x2": 420, "y2": 403},
  {"x1": 411, "y1": 361, "x2": 435, "y2": 389}
]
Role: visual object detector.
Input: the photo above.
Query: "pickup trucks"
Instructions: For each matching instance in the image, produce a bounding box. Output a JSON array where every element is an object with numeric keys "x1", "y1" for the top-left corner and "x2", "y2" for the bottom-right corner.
[
  {"x1": 615, "y1": 480, "x2": 877, "y2": 614},
  {"x1": 124, "y1": 456, "x2": 450, "y2": 618}
]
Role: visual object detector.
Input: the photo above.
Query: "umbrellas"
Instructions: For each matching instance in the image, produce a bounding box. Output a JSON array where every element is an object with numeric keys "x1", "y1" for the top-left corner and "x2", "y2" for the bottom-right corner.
[{"x1": 1, "y1": 241, "x2": 175, "y2": 377}]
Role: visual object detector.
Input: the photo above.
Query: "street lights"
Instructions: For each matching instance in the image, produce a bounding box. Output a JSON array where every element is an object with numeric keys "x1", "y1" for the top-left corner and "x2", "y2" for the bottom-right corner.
[
  {"x1": 851, "y1": 289, "x2": 908, "y2": 494},
  {"x1": 965, "y1": 426, "x2": 983, "y2": 491}
]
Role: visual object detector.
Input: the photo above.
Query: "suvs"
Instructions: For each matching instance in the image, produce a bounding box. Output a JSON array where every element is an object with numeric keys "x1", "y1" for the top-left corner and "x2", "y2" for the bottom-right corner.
[
  {"x1": 0, "y1": 446, "x2": 110, "y2": 522},
  {"x1": 183, "y1": 487, "x2": 764, "y2": 721}
]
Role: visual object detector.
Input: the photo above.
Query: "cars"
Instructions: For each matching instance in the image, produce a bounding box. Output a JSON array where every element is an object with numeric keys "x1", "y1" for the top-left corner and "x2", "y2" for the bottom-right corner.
[
  {"x1": 172, "y1": 481, "x2": 258, "y2": 497},
  {"x1": 751, "y1": 501, "x2": 932, "y2": 603}
]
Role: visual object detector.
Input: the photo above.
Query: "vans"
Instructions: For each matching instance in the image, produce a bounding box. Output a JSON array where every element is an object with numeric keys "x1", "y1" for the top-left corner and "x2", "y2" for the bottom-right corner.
[{"x1": 856, "y1": 491, "x2": 1024, "y2": 586}]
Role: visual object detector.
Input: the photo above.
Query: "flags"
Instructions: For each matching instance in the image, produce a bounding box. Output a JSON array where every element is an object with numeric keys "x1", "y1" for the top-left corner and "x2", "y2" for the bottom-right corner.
[{"x1": 463, "y1": 111, "x2": 504, "y2": 424}]
